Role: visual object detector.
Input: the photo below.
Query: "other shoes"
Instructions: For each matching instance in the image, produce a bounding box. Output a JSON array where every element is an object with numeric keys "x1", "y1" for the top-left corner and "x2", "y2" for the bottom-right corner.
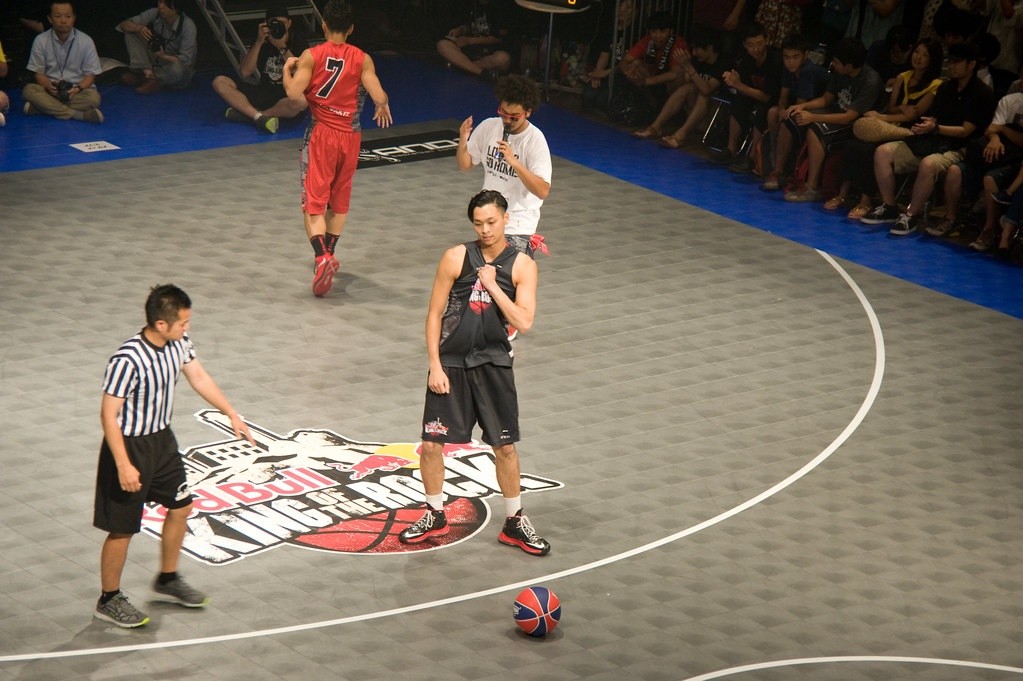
[
  {"x1": 256, "y1": 116, "x2": 279, "y2": 133},
  {"x1": 761, "y1": 171, "x2": 824, "y2": 201},
  {"x1": 656, "y1": 134, "x2": 685, "y2": 148},
  {"x1": 23, "y1": 102, "x2": 42, "y2": 115},
  {"x1": 632, "y1": 126, "x2": 661, "y2": 139},
  {"x1": 708, "y1": 148, "x2": 756, "y2": 173},
  {"x1": 824, "y1": 194, "x2": 875, "y2": 218},
  {"x1": 480, "y1": 69, "x2": 499, "y2": 82},
  {"x1": 84, "y1": 108, "x2": 103, "y2": 123},
  {"x1": 225, "y1": 108, "x2": 250, "y2": 123},
  {"x1": 134, "y1": 73, "x2": 161, "y2": 94}
]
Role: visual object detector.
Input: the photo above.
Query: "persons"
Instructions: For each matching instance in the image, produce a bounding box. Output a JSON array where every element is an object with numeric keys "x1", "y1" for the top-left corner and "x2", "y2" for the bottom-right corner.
[
  {"x1": 0, "y1": 41, "x2": 9, "y2": 127},
  {"x1": 283, "y1": 0, "x2": 393, "y2": 295},
  {"x1": 456, "y1": 74, "x2": 554, "y2": 341},
  {"x1": 212, "y1": 0, "x2": 308, "y2": 134},
  {"x1": 438, "y1": 0, "x2": 511, "y2": 81},
  {"x1": 17, "y1": 0, "x2": 103, "y2": 124},
  {"x1": 94, "y1": 285, "x2": 256, "y2": 627},
  {"x1": 590, "y1": 0, "x2": 1023, "y2": 258},
  {"x1": 120, "y1": 0, "x2": 197, "y2": 94},
  {"x1": 399, "y1": 188, "x2": 551, "y2": 557}
]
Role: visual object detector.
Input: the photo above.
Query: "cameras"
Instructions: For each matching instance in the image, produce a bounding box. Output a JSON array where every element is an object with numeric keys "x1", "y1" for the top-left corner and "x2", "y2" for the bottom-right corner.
[
  {"x1": 57, "y1": 80, "x2": 73, "y2": 103},
  {"x1": 262, "y1": 19, "x2": 286, "y2": 39},
  {"x1": 149, "y1": 34, "x2": 166, "y2": 52}
]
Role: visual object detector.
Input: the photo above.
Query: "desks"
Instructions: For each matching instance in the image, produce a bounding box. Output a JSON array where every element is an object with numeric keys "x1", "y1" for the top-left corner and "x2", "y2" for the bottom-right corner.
[{"x1": 515, "y1": 0, "x2": 591, "y2": 102}]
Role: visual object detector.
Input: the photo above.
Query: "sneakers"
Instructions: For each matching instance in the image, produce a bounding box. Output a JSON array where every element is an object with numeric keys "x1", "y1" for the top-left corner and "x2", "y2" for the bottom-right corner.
[
  {"x1": 969, "y1": 226, "x2": 995, "y2": 250},
  {"x1": 153, "y1": 575, "x2": 209, "y2": 607},
  {"x1": 399, "y1": 501, "x2": 449, "y2": 543},
  {"x1": 923, "y1": 220, "x2": 960, "y2": 237},
  {"x1": 991, "y1": 190, "x2": 1012, "y2": 204},
  {"x1": 312, "y1": 252, "x2": 339, "y2": 295},
  {"x1": 498, "y1": 508, "x2": 551, "y2": 555},
  {"x1": 860, "y1": 202, "x2": 919, "y2": 235},
  {"x1": 95, "y1": 591, "x2": 151, "y2": 628}
]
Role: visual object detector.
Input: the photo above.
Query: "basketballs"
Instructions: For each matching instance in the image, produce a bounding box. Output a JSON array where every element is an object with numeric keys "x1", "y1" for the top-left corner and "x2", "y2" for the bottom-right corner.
[{"x1": 512, "y1": 587, "x2": 561, "y2": 637}]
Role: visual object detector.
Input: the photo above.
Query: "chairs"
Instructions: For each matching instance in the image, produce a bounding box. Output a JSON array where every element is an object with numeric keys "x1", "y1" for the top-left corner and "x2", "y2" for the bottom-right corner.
[{"x1": 699, "y1": 91, "x2": 753, "y2": 156}]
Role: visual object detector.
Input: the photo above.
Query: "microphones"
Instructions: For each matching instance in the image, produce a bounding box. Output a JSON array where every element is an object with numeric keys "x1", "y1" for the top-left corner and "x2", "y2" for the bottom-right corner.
[{"x1": 498, "y1": 124, "x2": 511, "y2": 162}]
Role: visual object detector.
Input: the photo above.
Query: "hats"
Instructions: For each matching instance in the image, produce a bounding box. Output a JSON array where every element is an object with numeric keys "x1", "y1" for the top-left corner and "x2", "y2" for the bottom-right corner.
[{"x1": 940, "y1": 42, "x2": 980, "y2": 62}]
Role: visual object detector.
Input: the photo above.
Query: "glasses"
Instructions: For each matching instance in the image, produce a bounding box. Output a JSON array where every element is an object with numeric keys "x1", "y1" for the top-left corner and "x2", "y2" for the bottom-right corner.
[{"x1": 498, "y1": 105, "x2": 525, "y2": 121}]
